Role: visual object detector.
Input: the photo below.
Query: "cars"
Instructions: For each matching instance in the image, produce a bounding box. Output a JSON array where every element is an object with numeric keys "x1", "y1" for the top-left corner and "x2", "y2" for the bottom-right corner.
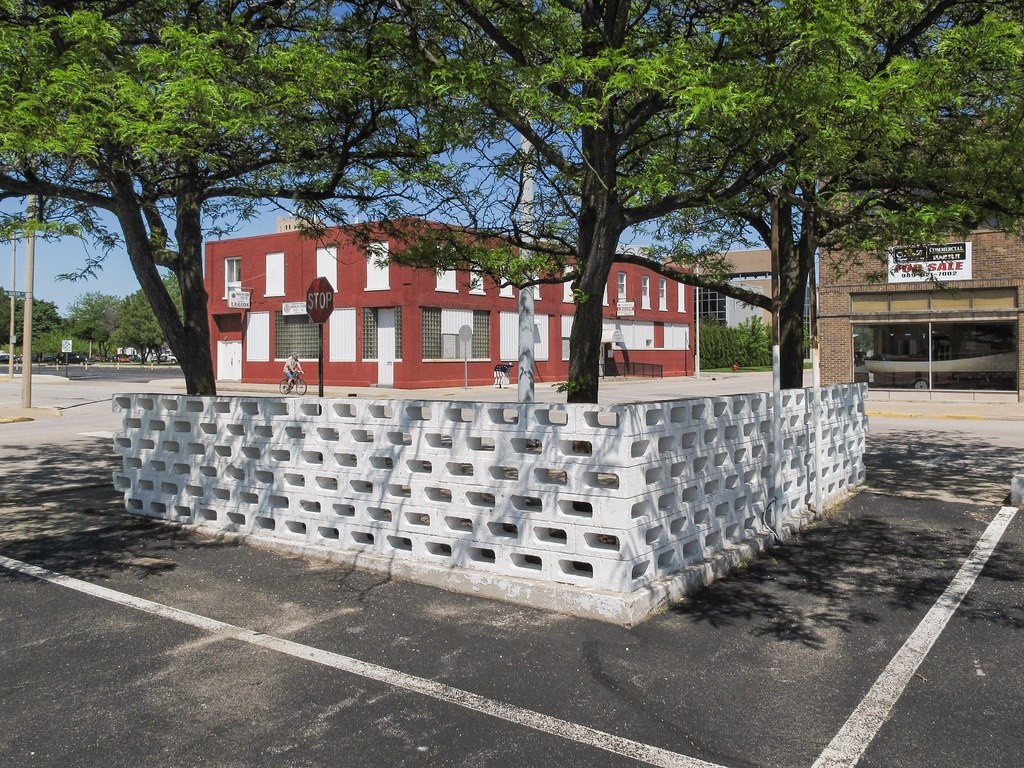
[{"x1": 1, "y1": 351, "x2": 178, "y2": 365}]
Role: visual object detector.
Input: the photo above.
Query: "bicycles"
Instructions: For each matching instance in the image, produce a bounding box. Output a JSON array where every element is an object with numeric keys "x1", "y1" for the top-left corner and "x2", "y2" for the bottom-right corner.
[{"x1": 279, "y1": 369, "x2": 307, "y2": 395}]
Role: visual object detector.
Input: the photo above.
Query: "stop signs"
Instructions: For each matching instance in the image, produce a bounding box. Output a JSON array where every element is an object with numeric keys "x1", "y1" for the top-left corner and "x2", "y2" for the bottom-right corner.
[{"x1": 305, "y1": 276, "x2": 335, "y2": 323}]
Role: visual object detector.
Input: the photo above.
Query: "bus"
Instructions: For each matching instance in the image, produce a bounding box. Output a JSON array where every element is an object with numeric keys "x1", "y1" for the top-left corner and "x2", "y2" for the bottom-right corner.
[{"x1": 865, "y1": 322, "x2": 1019, "y2": 388}]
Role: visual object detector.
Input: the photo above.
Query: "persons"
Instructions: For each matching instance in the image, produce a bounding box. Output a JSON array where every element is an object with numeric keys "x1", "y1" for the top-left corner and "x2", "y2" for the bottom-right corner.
[{"x1": 283, "y1": 352, "x2": 305, "y2": 393}]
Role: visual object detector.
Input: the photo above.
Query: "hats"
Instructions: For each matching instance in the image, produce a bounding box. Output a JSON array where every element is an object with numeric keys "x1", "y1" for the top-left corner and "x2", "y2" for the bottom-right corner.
[{"x1": 291, "y1": 352, "x2": 301, "y2": 356}]
